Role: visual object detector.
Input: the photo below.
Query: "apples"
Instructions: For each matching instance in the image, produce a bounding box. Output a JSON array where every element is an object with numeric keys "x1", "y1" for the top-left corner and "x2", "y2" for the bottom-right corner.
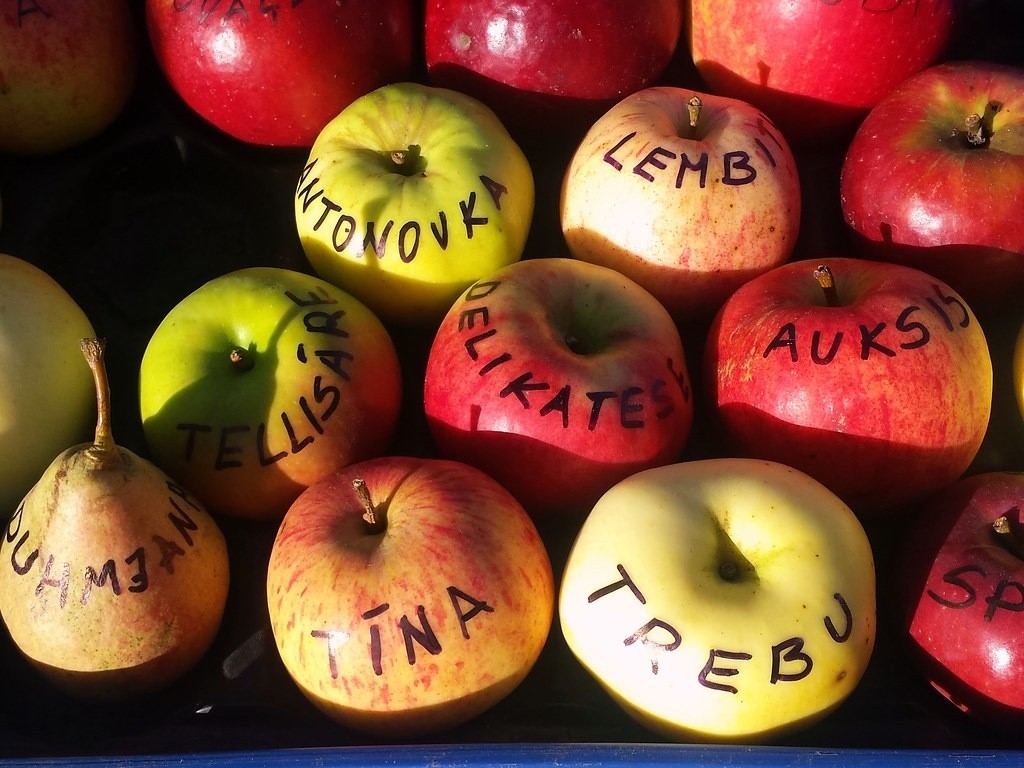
[{"x1": 0, "y1": 0, "x2": 1024, "y2": 745}]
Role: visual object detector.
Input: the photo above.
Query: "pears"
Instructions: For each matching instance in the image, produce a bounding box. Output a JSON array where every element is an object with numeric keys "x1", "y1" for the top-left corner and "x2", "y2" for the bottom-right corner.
[{"x1": 0, "y1": 333, "x2": 232, "y2": 704}]
